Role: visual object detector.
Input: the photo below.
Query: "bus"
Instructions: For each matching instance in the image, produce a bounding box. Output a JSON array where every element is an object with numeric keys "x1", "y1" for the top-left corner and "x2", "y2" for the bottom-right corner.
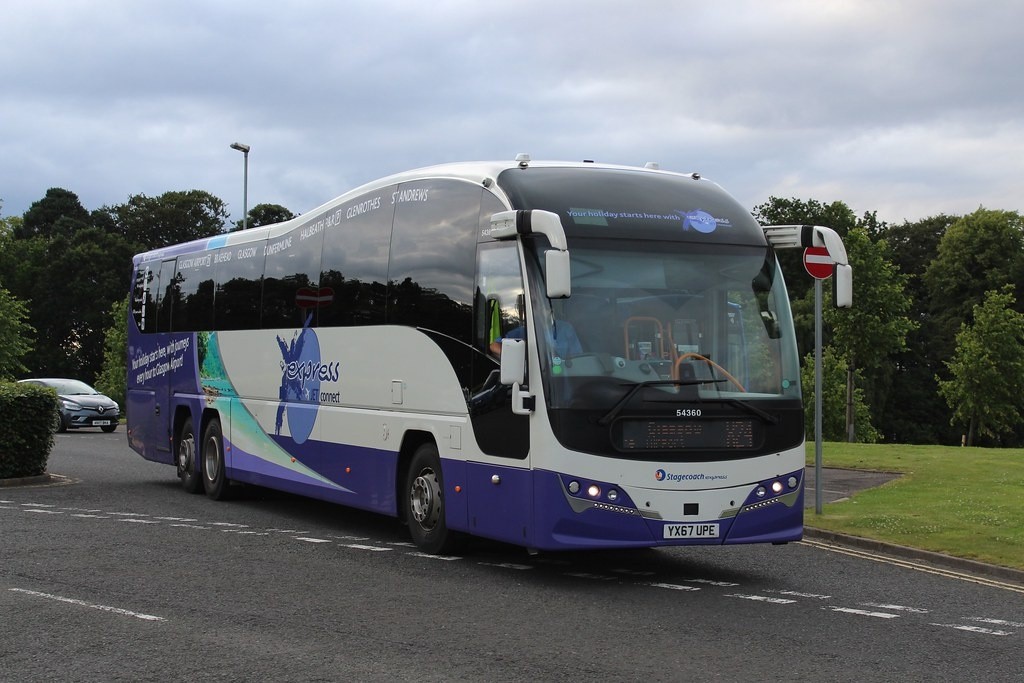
[{"x1": 124, "y1": 152, "x2": 854, "y2": 557}]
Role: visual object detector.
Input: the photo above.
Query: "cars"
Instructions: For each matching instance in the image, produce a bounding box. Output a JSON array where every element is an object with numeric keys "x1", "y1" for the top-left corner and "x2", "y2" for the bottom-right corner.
[{"x1": 17, "y1": 378, "x2": 121, "y2": 434}]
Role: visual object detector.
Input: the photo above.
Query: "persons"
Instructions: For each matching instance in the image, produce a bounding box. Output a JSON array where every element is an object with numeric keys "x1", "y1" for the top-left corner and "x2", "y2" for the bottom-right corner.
[{"x1": 489, "y1": 299, "x2": 585, "y2": 407}]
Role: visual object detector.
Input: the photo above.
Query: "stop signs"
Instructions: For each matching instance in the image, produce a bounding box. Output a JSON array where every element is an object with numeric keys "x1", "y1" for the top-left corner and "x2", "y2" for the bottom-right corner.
[
  {"x1": 316, "y1": 287, "x2": 334, "y2": 308},
  {"x1": 296, "y1": 287, "x2": 316, "y2": 306},
  {"x1": 802, "y1": 242, "x2": 838, "y2": 279}
]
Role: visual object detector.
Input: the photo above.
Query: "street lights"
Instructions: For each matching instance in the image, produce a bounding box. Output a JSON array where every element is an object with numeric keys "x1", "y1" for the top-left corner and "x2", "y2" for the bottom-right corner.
[{"x1": 230, "y1": 142, "x2": 252, "y2": 230}]
